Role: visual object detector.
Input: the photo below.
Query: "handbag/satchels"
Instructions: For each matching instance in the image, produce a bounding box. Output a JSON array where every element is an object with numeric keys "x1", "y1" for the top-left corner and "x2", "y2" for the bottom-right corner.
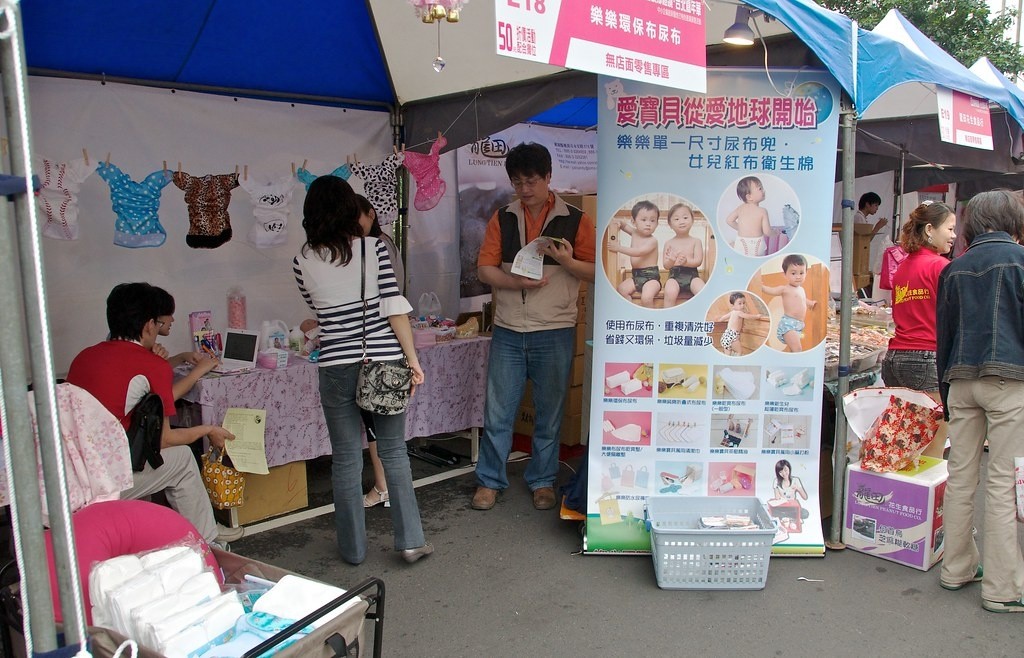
[
  {"x1": 1014, "y1": 456, "x2": 1024, "y2": 522},
  {"x1": 201, "y1": 444, "x2": 246, "y2": 510},
  {"x1": 842, "y1": 386, "x2": 946, "y2": 473},
  {"x1": 355, "y1": 362, "x2": 415, "y2": 416},
  {"x1": 127, "y1": 392, "x2": 164, "y2": 472}
]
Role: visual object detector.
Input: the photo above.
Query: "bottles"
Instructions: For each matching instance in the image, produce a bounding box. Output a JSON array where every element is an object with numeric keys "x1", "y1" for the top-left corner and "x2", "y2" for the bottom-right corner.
[
  {"x1": 289, "y1": 326, "x2": 304, "y2": 352},
  {"x1": 259, "y1": 320, "x2": 289, "y2": 353}
]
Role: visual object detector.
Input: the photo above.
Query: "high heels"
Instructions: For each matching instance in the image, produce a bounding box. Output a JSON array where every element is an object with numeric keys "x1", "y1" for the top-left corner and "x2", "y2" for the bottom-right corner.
[{"x1": 363, "y1": 485, "x2": 391, "y2": 508}]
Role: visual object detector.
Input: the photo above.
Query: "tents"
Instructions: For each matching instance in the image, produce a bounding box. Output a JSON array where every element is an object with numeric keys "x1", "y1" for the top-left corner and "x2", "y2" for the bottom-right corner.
[{"x1": 1, "y1": 0, "x2": 854, "y2": 658}]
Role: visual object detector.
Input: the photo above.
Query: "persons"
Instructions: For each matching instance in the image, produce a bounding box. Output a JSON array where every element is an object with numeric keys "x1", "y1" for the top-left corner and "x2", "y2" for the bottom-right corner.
[
  {"x1": 773, "y1": 459, "x2": 809, "y2": 527},
  {"x1": 470, "y1": 142, "x2": 596, "y2": 511},
  {"x1": 66, "y1": 282, "x2": 244, "y2": 554},
  {"x1": 606, "y1": 199, "x2": 661, "y2": 309},
  {"x1": 152, "y1": 285, "x2": 218, "y2": 401},
  {"x1": 881, "y1": 199, "x2": 956, "y2": 405},
  {"x1": 759, "y1": 255, "x2": 816, "y2": 353},
  {"x1": 714, "y1": 292, "x2": 762, "y2": 357},
  {"x1": 662, "y1": 203, "x2": 706, "y2": 308},
  {"x1": 725, "y1": 176, "x2": 772, "y2": 256},
  {"x1": 290, "y1": 173, "x2": 435, "y2": 567},
  {"x1": 935, "y1": 191, "x2": 1024, "y2": 613},
  {"x1": 853, "y1": 191, "x2": 888, "y2": 298}
]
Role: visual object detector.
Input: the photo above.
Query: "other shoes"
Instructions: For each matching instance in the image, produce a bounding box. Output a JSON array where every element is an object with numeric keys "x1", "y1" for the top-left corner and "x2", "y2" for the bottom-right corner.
[
  {"x1": 533, "y1": 487, "x2": 556, "y2": 510},
  {"x1": 471, "y1": 485, "x2": 499, "y2": 510},
  {"x1": 401, "y1": 541, "x2": 434, "y2": 563},
  {"x1": 982, "y1": 599, "x2": 1024, "y2": 613},
  {"x1": 213, "y1": 522, "x2": 244, "y2": 544},
  {"x1": 940, "y1": 565, "x2": 983, "y2": 590}
]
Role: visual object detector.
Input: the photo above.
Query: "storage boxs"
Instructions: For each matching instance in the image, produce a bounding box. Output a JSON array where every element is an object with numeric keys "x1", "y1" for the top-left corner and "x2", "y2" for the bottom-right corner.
[
  {"x1": 842, "y1": 455, "x2": 950, "y2": 571},
  {"x1": 647, "y1": 498, "x2": 779, "y2": 591},
  {"x1": 833, "y1": 222, "x2": 873, "y2": 289},
  {"x1": 216, "y1": 460, "x2": 309, "y2": 525},
  {"x1": 823, "y1": 306, "x2": 892, "y2": 382},
  {"x1": 491, "y1": 192, "x2": 597, "y2": 446},
  {"x1": 188, "y1": 310, "x2": 212, "y2": 354}
]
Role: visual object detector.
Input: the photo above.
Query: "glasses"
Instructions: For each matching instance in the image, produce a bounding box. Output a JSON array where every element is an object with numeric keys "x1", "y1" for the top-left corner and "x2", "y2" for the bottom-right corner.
[
  {"x1": 510, "y1": 175, "x2": 544, "y2": 189},
  {"x1": 145, "y1": 317, "x2": 165, "y2": 328}
]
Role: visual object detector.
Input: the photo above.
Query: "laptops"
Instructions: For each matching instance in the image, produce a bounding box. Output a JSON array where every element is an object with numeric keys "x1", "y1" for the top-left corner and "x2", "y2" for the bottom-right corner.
[{"x1": 210, "y1": 328, "x2": 260, "y2": 373}]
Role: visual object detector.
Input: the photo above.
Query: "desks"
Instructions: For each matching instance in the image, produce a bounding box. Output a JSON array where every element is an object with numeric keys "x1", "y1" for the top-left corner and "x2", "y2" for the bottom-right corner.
[
  {"x1": 823, "y1": 366, "x2": 881, "y2": 448},
  {"x1": 172, "y1": 336, "x2": 490, "y2": 534}
]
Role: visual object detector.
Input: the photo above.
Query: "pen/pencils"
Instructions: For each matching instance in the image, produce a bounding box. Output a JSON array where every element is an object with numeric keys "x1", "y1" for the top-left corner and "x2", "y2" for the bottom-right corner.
[
  {"x1": 201, "y1": 338, "x2": 215, "y2": 352},
  {"x1": 201, "y1": 345, "x2": 216, "y2": 359},
  {"x1": 212, "y1": 335, "x2": 215, "y2": 348}
]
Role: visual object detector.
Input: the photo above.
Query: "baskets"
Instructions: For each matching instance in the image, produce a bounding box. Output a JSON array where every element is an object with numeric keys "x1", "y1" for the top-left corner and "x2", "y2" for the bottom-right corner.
[{"x1": 644, "y1": 495, "x2": 777, "y2": 591}]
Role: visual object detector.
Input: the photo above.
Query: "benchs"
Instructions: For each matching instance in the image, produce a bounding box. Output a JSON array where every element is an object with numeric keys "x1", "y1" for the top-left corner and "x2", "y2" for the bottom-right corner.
[{"x1": 619, "y1": 266, "x2": 694, "y2": 309}]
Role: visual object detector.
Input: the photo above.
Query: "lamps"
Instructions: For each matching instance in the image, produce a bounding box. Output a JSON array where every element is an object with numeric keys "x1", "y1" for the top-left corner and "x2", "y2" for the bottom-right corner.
[{"x1": 723, "y1": 4, "x2": 775, "y2": 44}]
